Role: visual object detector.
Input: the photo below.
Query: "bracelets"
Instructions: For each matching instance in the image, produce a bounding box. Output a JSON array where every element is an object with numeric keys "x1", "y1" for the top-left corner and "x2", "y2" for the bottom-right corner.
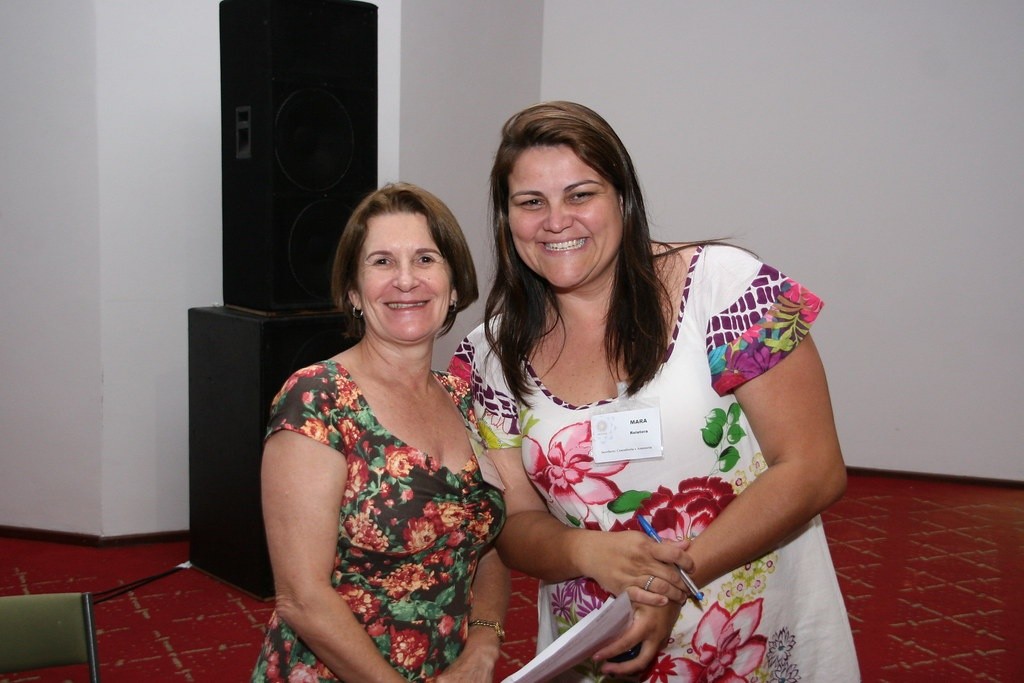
[{"x1": 469, "y1": 619, "x2": 505, "y2": 639}]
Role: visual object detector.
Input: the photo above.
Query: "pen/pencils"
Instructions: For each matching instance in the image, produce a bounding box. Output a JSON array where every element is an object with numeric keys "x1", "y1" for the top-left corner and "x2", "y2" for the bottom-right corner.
[{"x1": 633, "y1": 509, "x2": 707, "y2": 606}]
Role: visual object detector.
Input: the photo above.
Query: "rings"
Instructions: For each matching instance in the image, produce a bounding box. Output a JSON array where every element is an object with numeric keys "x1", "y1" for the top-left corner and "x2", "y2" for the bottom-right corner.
[{"x1": 645, "y1": 576, "x2": 654, "y2": 590}]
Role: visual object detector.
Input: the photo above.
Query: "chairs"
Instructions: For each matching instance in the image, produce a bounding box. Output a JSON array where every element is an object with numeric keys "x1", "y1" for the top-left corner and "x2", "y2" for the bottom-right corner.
[{"x1": 0, "y1": 592, "x2": 101, "y2": 683}]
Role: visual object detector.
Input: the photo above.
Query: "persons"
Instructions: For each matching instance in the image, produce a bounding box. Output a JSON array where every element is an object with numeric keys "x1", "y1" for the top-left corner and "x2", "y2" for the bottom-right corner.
[
  {"x1": 249, "y1": 183, "x2": 511, "y2": 683},
  {"x1": 448, "y1": 101, "x2": 862, "y2": 683}
]
